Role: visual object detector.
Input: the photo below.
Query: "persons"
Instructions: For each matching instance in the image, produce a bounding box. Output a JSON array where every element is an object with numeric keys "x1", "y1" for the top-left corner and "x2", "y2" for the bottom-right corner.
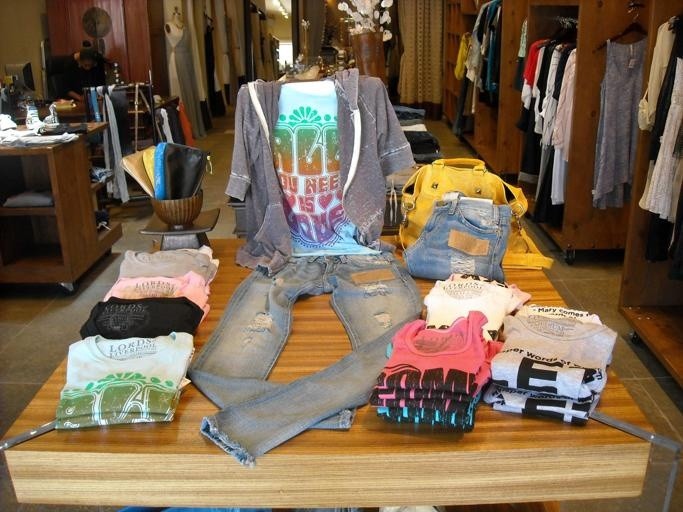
[
  {"x1": 53, "y1": 39, "x2": 105, "y2": 102},
  {"x1": 165, "y1": 12, "x2": 207, "y2": 139}
]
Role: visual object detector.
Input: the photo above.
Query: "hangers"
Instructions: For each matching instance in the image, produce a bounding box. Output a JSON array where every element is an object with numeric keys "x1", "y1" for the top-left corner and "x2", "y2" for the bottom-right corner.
[
  {"x1": 601, "y1": 1, "x2": 648, "y2": 49},
  {"x1": 536, "y1": 16, "x2": 578, "y2": 49}
]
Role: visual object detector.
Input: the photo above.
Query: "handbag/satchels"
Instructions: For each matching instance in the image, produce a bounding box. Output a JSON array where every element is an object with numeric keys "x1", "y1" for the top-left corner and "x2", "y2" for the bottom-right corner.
[
  {"x1": 163, "y1": 144, "x2": 213, "y2": 199},
  {"x1": 142, "y1": 147, "x2": 156, "y2": 191},
  {"x1": 153, "y1": 142, "x2": 170, "y2": 200},
  {"x1": 119, "y1": 150, "x2": 153, "y2": 200}
]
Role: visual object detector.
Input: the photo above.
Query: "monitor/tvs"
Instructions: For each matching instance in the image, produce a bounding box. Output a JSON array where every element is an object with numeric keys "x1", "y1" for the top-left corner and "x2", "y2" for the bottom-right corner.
[{"x1": 4, "y1": 62, "x2": 36, "y2": 91}]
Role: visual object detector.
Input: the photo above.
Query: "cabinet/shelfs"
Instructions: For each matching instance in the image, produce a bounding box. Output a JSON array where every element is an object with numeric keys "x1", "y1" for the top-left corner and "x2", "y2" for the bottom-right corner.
[
  {"x1": 518, "y1": 0, "x2": 648, "y2": 265},
  {"x1": 617, "y1": 1, "x2": 683, "y2": 387},
  {"x1": 0, "y1": 122, "x2": 123, "y2": 292},
  {"x1": 443, "y1": 0, "x2": 503, "y2": 175}
]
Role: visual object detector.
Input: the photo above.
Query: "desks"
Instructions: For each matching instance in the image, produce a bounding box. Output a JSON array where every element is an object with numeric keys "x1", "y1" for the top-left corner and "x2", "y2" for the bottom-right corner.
[{"x1": 1, "y1": 235, "x2": 655, "y2": 510}]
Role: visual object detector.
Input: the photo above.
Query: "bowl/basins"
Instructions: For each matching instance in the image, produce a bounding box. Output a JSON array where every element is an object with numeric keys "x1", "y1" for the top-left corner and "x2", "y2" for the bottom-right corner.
[{"x1": 149, "y1": 189, "x2": 203, "y2": 226}]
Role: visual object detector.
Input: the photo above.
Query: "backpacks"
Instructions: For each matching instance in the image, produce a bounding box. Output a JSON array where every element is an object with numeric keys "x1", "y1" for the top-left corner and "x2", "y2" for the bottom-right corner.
[{"x1": 393, "y1": 157, "x2": 554, "y2": 270}]
